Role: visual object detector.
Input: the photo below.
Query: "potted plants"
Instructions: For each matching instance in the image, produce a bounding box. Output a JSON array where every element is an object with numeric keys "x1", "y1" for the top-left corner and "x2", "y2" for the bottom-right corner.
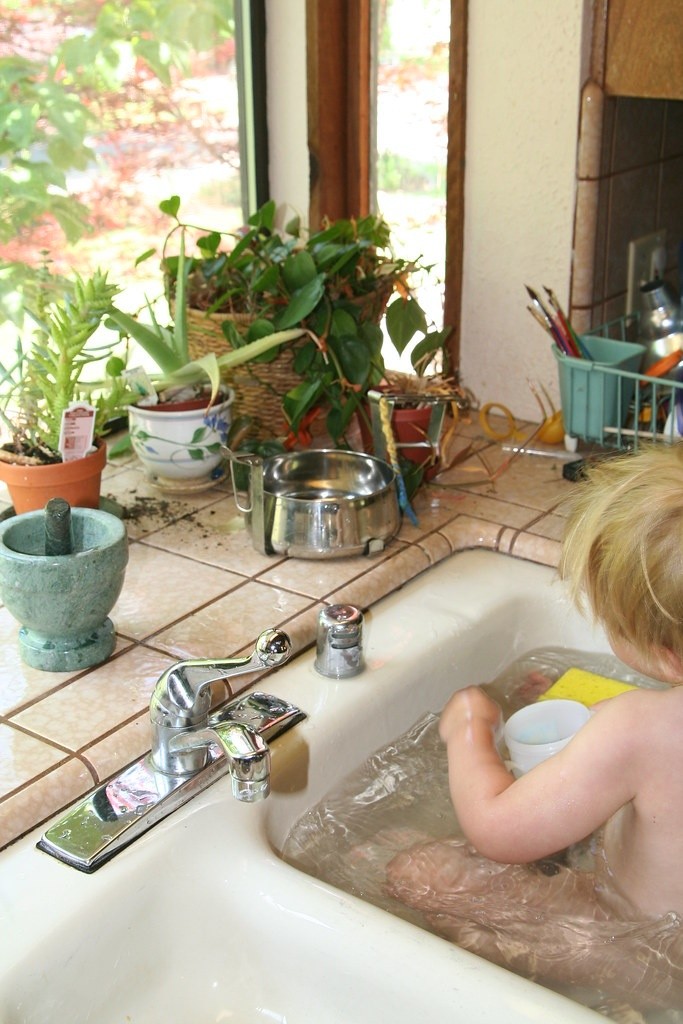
[
  {"x1": 133, "y1": 195, "x2": 454, "y2": 502},
  {"x1": 0, "y1": 265, "x2": 147, "y2": 515},
  {"x1": 104, "y1": 229, "x2": 329, "y2": 494},
  {"x1": 355, "y1": 378, "x2": 470, "y2": 483}
]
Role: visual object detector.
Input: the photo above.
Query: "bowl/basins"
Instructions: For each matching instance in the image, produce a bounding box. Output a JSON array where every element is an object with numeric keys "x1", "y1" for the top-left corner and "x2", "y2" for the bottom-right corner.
[{"x1": 217, "y1": 446, "x2": 402, "y2": 559}]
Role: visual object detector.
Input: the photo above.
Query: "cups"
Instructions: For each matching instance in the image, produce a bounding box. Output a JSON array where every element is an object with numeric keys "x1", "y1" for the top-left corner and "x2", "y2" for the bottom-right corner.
[
  {"x1": 503, "y1": 699, "x2": 592, "y2": 779},
  {"x1": 551, "y1": 335, "x2": 649, "y2": 440}
]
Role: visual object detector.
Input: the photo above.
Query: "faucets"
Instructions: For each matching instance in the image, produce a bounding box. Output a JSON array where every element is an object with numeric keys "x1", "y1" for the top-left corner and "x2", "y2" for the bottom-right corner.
[{"x1": 148, "y1": 629, "x2": 292, "y2": 801}]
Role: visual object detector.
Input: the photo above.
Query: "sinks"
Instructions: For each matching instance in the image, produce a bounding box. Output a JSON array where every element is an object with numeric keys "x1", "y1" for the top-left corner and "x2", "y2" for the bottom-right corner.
[
  {"x1": 237, "y1": 548, "x2": 682, "y2": 1024},
  {"x1": 0, "y1": 838, "x2": 622, "y2": 1024}
]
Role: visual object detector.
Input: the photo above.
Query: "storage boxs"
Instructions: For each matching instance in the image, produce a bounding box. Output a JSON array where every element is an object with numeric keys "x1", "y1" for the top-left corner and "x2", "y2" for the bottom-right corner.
[{"x1": 551, "y1": 336, "x2": 647, "y2": 441}]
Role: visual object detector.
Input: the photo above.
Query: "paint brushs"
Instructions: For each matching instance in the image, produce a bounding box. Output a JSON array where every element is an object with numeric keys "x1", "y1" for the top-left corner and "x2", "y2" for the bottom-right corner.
[{"x1": 522, "y1": 282, "x2": 593, "y2": 361}]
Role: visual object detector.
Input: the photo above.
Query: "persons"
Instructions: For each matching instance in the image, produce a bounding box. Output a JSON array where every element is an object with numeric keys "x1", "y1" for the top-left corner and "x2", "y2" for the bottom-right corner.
[{"x1": 379, "y1": 436, "x2": 683, "y2": 965}]
